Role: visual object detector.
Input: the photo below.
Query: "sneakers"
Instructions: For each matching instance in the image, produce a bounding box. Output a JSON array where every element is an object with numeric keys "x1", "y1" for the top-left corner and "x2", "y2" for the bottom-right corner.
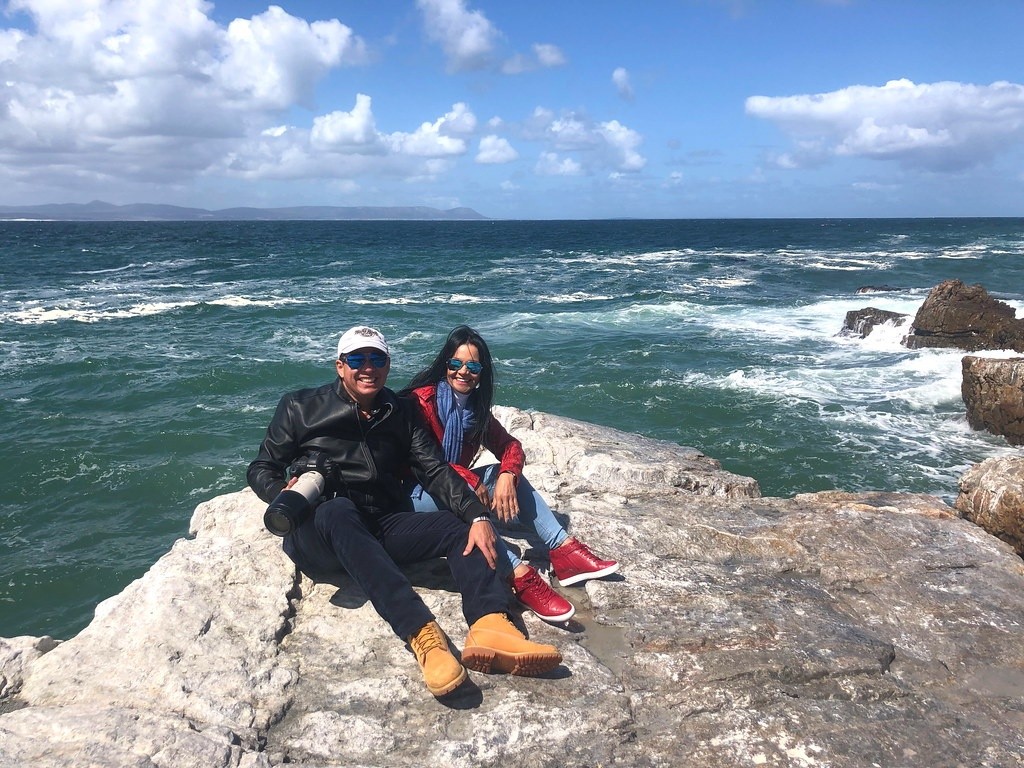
[
  {"x1": 407, "y1": 620, "x2": 467, "y2": 696},
  {"x1": 461, "y1": 613, "x2": 562, "y2": 676},
  {"x1": 549, "y1": 535, "x2": 619, "y2": 586},
  {"x1": 506, "y1": 565, "x2": 575, "y2": 622}
]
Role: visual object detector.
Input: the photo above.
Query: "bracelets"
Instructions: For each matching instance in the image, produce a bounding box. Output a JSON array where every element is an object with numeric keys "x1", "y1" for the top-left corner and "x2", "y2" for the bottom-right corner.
[
  {"x1": 507, "y1": 471, "x2": 516, "y2": 482},
  {"x1": 472, "y1": 516, "x2": 491, "y2": 523}
]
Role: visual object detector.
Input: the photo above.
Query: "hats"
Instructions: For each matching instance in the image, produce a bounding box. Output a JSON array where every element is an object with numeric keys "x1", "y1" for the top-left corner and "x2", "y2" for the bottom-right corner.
[{"x1": 338, "y1": 326, "x2": 389, "y2": 359}]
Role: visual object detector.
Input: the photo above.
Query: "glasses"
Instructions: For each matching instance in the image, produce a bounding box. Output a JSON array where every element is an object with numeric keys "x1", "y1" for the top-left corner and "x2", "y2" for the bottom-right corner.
[
  {"x1": 341, "y1": 352, "x2": 388, "y2": 368},
  {"x1": 445, "y1": 358, "x2": 483, "y2": 375}
]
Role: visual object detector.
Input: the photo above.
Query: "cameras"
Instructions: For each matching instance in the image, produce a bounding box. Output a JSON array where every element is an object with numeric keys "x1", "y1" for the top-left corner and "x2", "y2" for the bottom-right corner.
[{"x1": 264, "y1": 451, "x2": 351, "y2": 536}]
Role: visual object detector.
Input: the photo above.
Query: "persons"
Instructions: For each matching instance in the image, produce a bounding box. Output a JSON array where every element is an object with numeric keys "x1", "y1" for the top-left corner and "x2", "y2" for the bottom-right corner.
[
  {"x1": 392, "y1": 325, "x2": 622, "y2": 624},
  {"x1": 246, "y1": 324, "x2": 564, "y2": 696}
]
